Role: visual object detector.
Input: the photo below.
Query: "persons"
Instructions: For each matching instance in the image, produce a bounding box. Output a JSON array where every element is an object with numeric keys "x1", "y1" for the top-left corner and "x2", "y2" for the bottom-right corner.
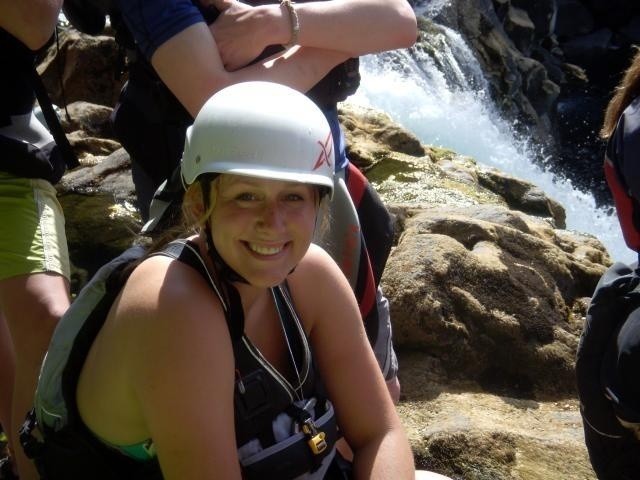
[
  {"x1": 62, "y1": 0, "x2": 137, "y2": 71},
  {"x1": 576, "y1": 49, "x2": 639, "y2": 480},
  {"x1": 1, "y1": 1, "x2": 79, "y2": 478},
  {"x1": 99, "y1": 0, "x2": 421, "y2": 411},
  {"x1": 24, "y1": 80, "x2": 457, "y2": 480}
]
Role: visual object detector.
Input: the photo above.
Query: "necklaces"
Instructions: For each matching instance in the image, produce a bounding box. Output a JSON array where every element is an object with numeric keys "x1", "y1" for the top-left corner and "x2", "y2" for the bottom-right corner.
[{"x1": 228, "y1": 283, "x2": 327, "y2": 456}]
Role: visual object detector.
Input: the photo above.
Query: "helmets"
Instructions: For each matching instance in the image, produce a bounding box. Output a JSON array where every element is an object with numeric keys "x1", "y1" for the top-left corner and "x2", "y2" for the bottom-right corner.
[{"x1": 180, "y1": 79, "x2": 335, "y2": 204}]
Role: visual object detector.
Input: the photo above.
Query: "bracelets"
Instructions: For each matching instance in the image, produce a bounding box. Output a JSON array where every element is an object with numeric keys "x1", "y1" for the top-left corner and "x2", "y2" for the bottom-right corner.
[{"x1": 277, "y1": 0, "x2": 299, "y2": 53}]
